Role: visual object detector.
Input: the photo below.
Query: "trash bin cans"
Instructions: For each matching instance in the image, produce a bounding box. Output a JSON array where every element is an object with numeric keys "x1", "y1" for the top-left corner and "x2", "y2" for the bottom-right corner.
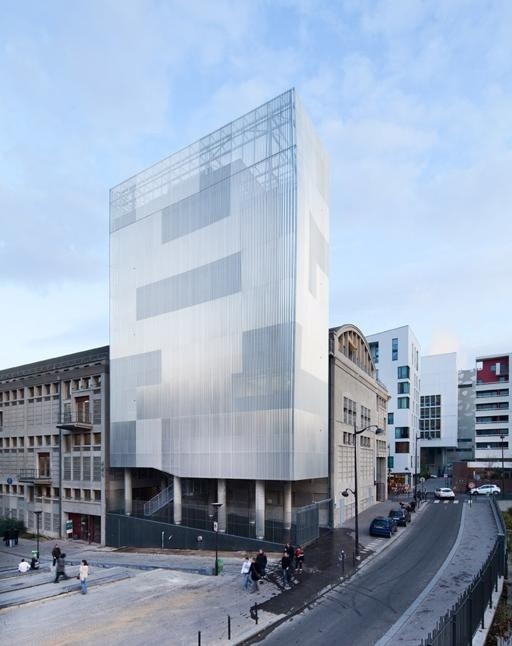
[
  {"x1": 215, "y1": 559, "x2": 224, "y2": 576},
  {"x1": 32, "y1": 551, "x2": 38, "y2": 560}
]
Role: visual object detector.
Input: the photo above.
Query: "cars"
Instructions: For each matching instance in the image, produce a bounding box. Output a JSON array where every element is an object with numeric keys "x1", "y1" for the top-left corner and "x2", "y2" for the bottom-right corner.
[{"x1": 434, "y1": 487, "x2": 456, "y2": 499}]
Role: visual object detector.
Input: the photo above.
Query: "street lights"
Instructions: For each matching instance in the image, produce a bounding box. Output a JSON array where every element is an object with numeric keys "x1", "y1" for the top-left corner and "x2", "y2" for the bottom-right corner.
[
  {"x1": 415, "y1": 431, "x2": 432, "y2": 501},
  {"x1": 212, "y1": 501, "x2": 224, "y2": 576},
  {"x1": 33, "y1": 510, "x2": 43, "y2": 561},
  {"x1": 343, "y1": 424, "x2": 385, "y2": 560},
  {"x1": 499, "y1": 432, "x2": 506, "y2": 495}
]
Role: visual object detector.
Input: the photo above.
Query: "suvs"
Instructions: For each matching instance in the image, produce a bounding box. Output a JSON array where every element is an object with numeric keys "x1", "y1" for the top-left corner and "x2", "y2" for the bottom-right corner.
[
  {"x1": 369, "y1": 515, "x2": 397, "y2": 537},
  {"x1": 469, "y1": 483, "x2": 501, "y2": 496},
  {"x1": 389, "y1": 508, "x2": 412, "y2": 527}
]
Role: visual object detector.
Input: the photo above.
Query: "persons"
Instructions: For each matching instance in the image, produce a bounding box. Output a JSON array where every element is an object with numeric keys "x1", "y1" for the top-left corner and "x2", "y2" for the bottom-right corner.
[
  {"x1": 54, "y1": 552, "x2": 71, "y2": 584},
  {"x1": 52, "y1": 543, "x2": 62, "y2": 567},
  {"x1": 31, "y1": 558, "x2": 41, "y2": 571},
  {"x1": 18, "y1": 559, "x2": 32, "y2": 573},
  {"x1": 240, "y1": 541, "x2": 305, "y2": 595},
  {"x1": 79, "y1": 560, "x2": 90, "y2": 595}
]
